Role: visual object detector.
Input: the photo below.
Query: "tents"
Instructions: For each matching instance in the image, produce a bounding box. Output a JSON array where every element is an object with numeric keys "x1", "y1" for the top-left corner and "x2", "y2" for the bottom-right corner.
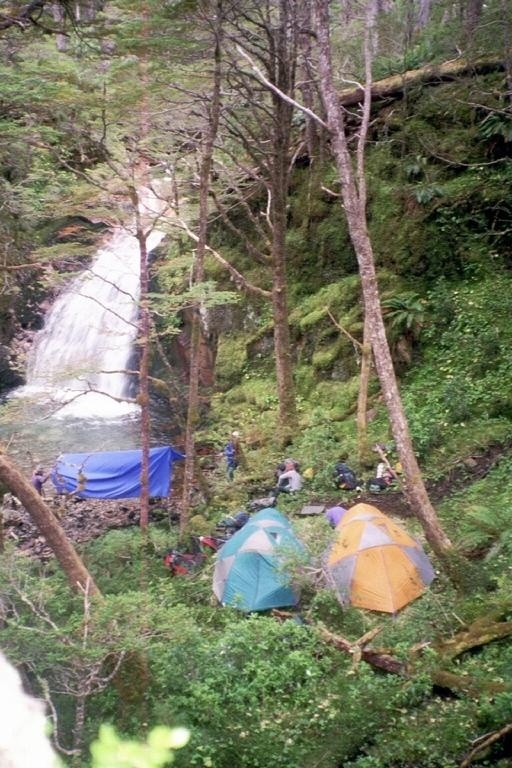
[
  {"x1": 212, "y1": 507, "x2": 310, "y2": 615},
  {"x1": 321, "y1": 503, "x2": 436, "y2": 616}
]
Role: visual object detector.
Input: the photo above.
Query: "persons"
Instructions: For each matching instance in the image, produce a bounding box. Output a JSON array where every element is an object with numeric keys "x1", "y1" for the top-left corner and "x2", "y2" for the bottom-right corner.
[
  {"x1": 29, "y1": 466, "x2": 52, "y2": 496},
  {"x1": 269, "y1": 459, "x2": 303, "y2": 501},
  {"x1": 223, "y1": 431, "x2": 241, "y2": 481}
]
